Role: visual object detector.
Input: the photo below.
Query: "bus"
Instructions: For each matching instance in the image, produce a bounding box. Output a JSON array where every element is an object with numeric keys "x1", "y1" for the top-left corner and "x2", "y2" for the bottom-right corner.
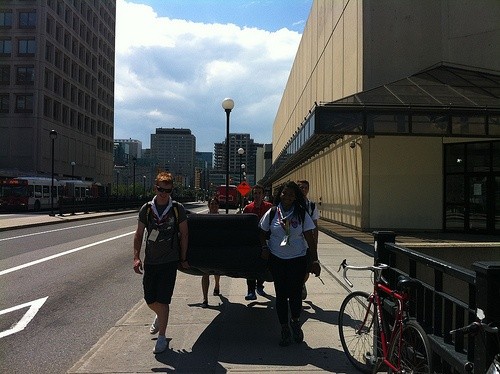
[
  {"x1": 0, "y1": 177, "x2": 99, "y2": 212},
  {"x1": 216, "y1": 184, "x2": 241, "y2": 209}
]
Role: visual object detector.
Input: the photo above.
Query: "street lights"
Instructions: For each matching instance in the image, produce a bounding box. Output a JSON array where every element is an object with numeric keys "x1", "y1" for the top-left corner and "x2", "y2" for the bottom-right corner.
[
  {"x1": 237, "y1": 148, "x2": 244, "y2": 213},
  {"x1": 71, "y1": 162, "x2": 75, "y2": 180},
  {"x1": 222, "y1": 98, "x2": 235, "y2": 214},
  {"x1": 132, "y1": 157, "x2": 137, "y2": 189},
  {"x1": 241, "y1": 163, "x2": 247, "y2": 210},
  {"x1": 48, "y1": 130, "x2": 57, "y2": 216},
  {"x1": 116, "y1": 171, "x2": 120, "y2": 195}
]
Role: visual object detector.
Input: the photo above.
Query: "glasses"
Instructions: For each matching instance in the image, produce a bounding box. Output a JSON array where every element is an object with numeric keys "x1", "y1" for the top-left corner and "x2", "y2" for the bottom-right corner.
[{"x1": 157, "y1": 187, "x2": 171, "y2": 193}]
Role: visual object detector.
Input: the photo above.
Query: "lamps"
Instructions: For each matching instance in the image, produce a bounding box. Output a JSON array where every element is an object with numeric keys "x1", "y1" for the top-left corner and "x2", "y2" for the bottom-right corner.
[{"x1": 349, "y1": 140, "x2": 360, "y2": 149}]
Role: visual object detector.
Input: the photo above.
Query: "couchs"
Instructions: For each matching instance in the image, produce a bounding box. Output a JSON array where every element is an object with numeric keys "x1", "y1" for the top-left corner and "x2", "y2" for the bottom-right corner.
[{"x1": 176, "y1": 214, "x2": 273, "y2": 282}]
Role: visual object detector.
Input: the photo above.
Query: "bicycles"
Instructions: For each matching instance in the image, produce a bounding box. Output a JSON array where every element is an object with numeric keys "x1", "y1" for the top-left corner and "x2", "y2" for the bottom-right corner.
[
  {"x1": 449, "y1": 306, "x2": 500, "y2": 374},
  {"x1": 338, "y1": 258, "x2": 433, "y2": 374}
]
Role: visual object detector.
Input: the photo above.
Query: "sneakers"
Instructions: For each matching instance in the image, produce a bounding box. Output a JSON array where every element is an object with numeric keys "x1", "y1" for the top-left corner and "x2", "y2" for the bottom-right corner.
[
  {"x1": 153, "y1": 336, "x2": 167, "y2": 354},
  {"x1": 150, "y1": 314, "x2": 159, "y2": 334}
]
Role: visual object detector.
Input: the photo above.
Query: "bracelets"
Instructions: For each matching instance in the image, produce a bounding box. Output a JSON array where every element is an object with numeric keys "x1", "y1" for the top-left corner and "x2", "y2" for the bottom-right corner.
[
  {"x1": 312, "y1": 260, "x2": 320, "y2": 263},
  {"x1": 180, "y1": 259, "x2": 187, "y2": 262}
]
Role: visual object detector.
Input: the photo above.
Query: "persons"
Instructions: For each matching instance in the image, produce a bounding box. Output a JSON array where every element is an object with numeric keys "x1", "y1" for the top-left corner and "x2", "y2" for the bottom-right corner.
[
  {"x1": 243, "y1": 184, "x2": 274, "y2": 300},
  {"x1": 202, "y1": 196, "x2": 220, "y2": 308},
  {"x1": 133, "y1": 171, "x2": 189, "y2": 353},
  {"x1": 258, "y1": 180, "x2": 321, "y2": 346}
]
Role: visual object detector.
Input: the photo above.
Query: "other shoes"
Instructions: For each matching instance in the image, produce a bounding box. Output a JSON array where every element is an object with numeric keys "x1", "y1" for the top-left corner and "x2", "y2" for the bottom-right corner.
[
  {"x1": 302, "y1": 284, "x2": 307, "y2": 300},
  {"x1": 202, "y1": 301, "x2": 208, "y2": 307},
  {"x1": 245, "y1": 293, "x2": 257, "y2": 300},
  {"x1": 280, "y1": 327, "x2": 291, "y2": 346},
  {"x1": 213, "y1": 287, "x2": 219, "y2": 296},
  {"x1": 290, "y1": 318, "x2": 304, "y2": 343},
  {"x1": 257, "y1": 290, "x2": 265, "y2": 296}
]
